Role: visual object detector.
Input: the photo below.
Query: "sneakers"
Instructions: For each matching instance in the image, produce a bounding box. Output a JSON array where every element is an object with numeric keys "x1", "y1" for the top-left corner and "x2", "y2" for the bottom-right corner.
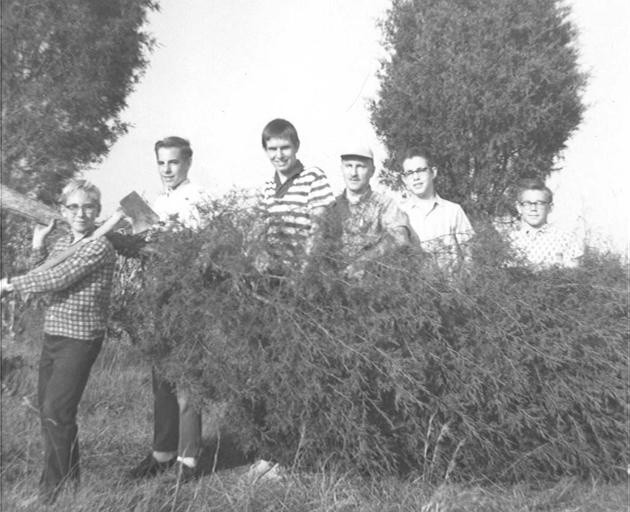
[
  {"x1": 125, "y1": 455, "x2": 198, "y2": 487},
  {"x1": 240, "y1": 459, "x2": 285, "y2": 487}
]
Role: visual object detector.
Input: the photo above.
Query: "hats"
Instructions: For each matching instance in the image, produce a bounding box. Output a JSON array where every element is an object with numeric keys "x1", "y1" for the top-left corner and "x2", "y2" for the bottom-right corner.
[{"x1": 340, "y1": 143, "x2": 375, "y2": 163}]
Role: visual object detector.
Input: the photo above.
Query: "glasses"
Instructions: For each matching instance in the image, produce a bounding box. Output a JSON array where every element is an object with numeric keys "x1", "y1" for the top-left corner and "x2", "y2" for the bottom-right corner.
[
  {"x1": 64, "y1": 202, "x2": 96, "y2": 214},
  {"x1": 519, "y1": 199, "x2": 548, "y2": 208},
  {"x1": 401, "y1": 166, "x2": 427, "y2": 176}
]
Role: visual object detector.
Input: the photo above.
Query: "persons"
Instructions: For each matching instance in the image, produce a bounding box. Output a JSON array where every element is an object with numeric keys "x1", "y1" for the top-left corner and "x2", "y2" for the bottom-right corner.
[
  {"x1": 235, "y1": 116, "x2": 334, "y2": 478},
  {"x1": 1, "y1": 179, "x2": 116, "y2": 502},
  {"x1": 395, "y1": 144, "x2": 476, "y2": 274},
  {"x1": 324, "y1": 135, "x2": 412, "y2": 282},
  {"x1": 502, "y1": 176, "x2": 583, "y2": 272},
  {"x1": 127, "y1": 134, "x2": 213, "y2": 482}
]
